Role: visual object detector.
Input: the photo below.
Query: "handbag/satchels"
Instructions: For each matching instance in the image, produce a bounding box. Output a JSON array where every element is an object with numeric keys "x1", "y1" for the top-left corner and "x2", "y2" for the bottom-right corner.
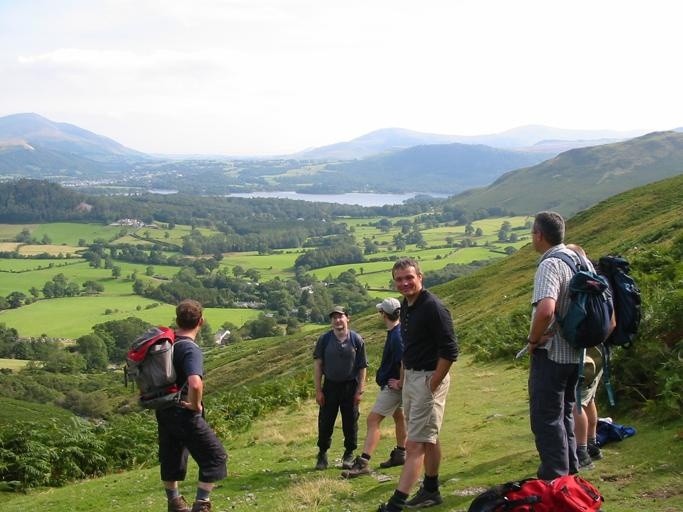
[{"x1": 376, "y1": 367, "x2": 387, "y2": 386}]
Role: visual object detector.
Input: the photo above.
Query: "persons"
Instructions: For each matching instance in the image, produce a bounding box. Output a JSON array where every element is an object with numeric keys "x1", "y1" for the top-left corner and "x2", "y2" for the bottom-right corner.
[
  {"x1": 153, "y1": 299, "x2": 229, "y2": 511},
  {"x1": 341, "y1": 296, "x2": 410, "y2": 479},
  {"x1": 375, "y1": 258, "x2": 460, "y2": 512},
  {"x1": 527, "y1": 210, "x2": 599, "y2": 481},
  {"x1": 565, "y1": 244, "x2": 609, "y2": 471},
  {"x1": 311, "y1": 304, "x2": 369, "y2": 469}
]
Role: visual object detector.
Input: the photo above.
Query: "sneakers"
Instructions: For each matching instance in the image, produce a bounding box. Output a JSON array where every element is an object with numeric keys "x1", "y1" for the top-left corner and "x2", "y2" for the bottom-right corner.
[
  {"x1": 193, "y1": 497, "x2": 211, "y2": 511},
  {"x1": 406, "y1": 485, "x2": 442, "y2": 508},
  {"x1": 577, "y1": 450, "x2": 592, "y2": 468},
  {"x1": 341, "y1": 449, "x2": 354, "y2": 469},
  {"x1": 341, "y1": 455, "x2": 370, "y2": 478},
  {"x1": 316, "y1": 452, "x2": 327, "y2": 470},
  {"x1": 168, "y1": 495, "x2": 192, "y2": 512},
  {"x1": 589, "y1": 441, "x2": 603, "y2": 459},
  {"x1": 380, "y1": 447, "x2": 406, "y2": 467}
]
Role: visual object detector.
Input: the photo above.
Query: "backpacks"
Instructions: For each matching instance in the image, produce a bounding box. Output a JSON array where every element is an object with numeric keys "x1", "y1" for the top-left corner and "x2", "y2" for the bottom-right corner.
[
  {"x1": 126, "y1": 327, "x2": 201, "y2": 410},
  {"x1": 468, "y1": 475, "x2": 604, "y2": 512},
  {"x1": 599, "y1": 255, "x2": 640, "y2": 346},
  {"x1": 546, "y1": 251, "x2": 617, "y2": 350}
]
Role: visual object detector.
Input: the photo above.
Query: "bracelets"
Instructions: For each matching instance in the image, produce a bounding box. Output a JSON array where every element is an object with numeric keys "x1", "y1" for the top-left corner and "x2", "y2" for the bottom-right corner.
[
  {"x1": 357, "y1": 390, "x2": 365, "y2": 395},
  {"x1": 527, "y1": 335, "x2": 538, "y2": 344}
]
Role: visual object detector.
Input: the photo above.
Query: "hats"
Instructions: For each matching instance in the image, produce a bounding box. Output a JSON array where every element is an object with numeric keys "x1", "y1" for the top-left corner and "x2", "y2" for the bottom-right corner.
[
  {"x1": 329, "y1": 306, "x2": 348, "y2": 316},
  {"x1": 376, "y1": 298, "x2": 401, "y2": 316}
]
